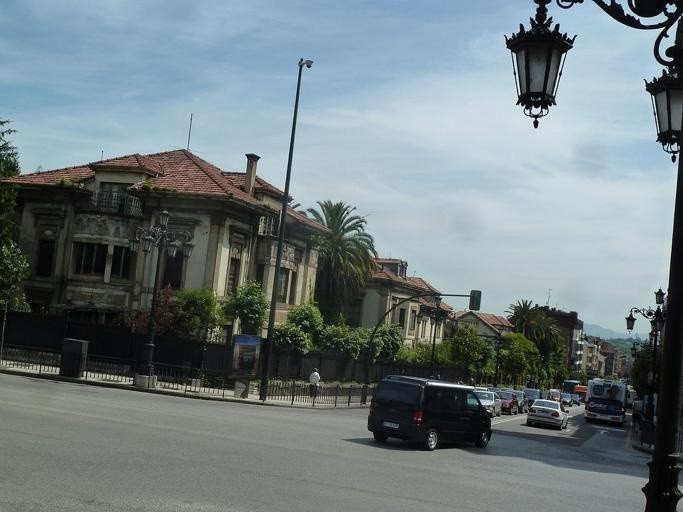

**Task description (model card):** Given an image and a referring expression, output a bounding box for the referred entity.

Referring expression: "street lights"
[133,210,183,385]
[256,54,317,401]
[623,281,670,451]
[502,0,683,511]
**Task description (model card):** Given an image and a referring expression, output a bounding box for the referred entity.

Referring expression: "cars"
[461,375,634,433]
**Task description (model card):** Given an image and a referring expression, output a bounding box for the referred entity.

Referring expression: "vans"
[365,374,493,451]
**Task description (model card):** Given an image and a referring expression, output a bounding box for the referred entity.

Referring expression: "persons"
[308,367,320,398]
